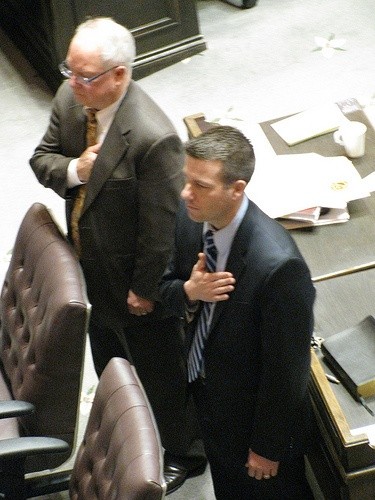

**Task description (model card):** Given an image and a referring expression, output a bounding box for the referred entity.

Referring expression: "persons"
[155,125,316,500]
[28,16,207,496]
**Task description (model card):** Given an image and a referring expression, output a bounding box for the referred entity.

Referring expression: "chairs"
[0,202,167,500]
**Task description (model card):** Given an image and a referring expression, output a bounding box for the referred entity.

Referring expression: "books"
[320,314,375,400]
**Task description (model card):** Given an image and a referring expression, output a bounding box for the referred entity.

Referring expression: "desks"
[181,94,375,500]
[0,0,208,98]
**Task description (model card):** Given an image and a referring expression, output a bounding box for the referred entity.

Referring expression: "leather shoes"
[162,464,205,496]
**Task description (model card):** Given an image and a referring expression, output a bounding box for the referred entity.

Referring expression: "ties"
[71,108,98,248]
[188,231,217,383]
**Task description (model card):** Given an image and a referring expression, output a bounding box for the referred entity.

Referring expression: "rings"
[263,475,270,479]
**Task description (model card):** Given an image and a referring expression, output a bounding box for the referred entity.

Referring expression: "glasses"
[57,62,118,87]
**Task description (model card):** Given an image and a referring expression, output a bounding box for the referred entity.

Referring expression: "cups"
[333,122,367,158]
